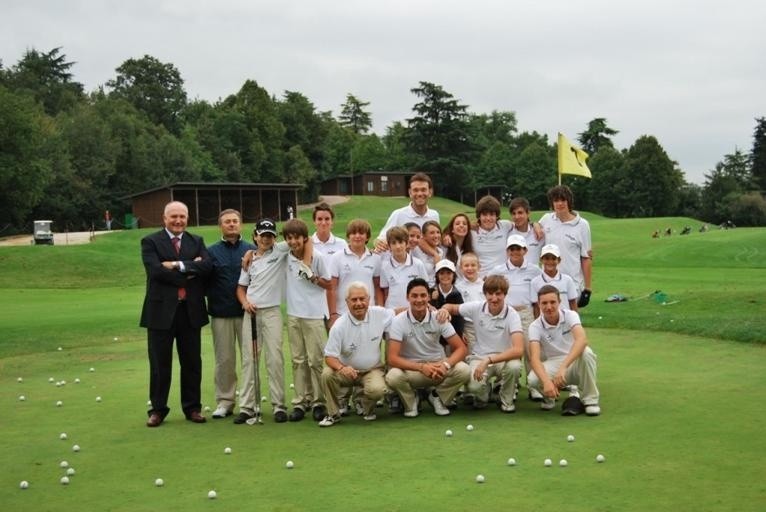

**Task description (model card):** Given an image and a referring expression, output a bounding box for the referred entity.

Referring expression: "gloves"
[577,288,591,308]
[299,262,314,281]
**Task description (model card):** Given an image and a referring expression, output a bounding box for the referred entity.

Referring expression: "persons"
[103,210,113,231]
[652,220,736,238]
[205,209,259,419]
[139,201,213,427]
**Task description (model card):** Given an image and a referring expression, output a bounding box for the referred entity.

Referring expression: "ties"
[172,238,188,302]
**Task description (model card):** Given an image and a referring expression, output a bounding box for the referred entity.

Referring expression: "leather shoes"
[146,413,161,427]
[190,411,205,424]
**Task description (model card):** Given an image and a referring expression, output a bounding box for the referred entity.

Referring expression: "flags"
[558,133,592,179]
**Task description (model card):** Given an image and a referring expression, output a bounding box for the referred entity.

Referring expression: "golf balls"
[59,433,81,485]
[286,461,293,468]
[20,481,29,489]
[467,424,473,430]
[476,474,484,483]
[446,430,452,436]
[57,347,63,351]
[148,400,152,405]
[208,490,216,498]
[225,448,231,454]
[670,320,674,323]
[508,434,606,466]
[598,317,602,319]
[656,312,660,316]
[156,479,164,486]
[17,367,103,408]
[290,383,295,389]
[262,396,267,402]
[114,337,118,341]
[205,406,211,411]
[236,391,239,396]
[662,302,666,305]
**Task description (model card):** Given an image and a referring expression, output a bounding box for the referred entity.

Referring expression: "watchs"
[172,261,177,269]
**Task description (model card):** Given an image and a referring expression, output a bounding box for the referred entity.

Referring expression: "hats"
[561,395,585,417]
[540,243,560,258]
[434,259,456,274]
[256,218,278,237]
[505,234,527,248]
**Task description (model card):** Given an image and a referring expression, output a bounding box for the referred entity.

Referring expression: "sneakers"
[273,411,288,423]
[388,397,402,414]
[561,385,581,397]
[403,389,420,418]
[584,405,601,416]
[540,396,556,410]
[318,407,347,427]
[288,408,304,422]
[312,406,326,420]
[451,385,519,413]
[211,407,230,418]
[232,412,250,424]
[355,401,383,420]
[527,387,544,400]
[427,389,450,416]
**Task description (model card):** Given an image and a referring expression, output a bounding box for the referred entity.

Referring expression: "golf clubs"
[246,311,264,426]
